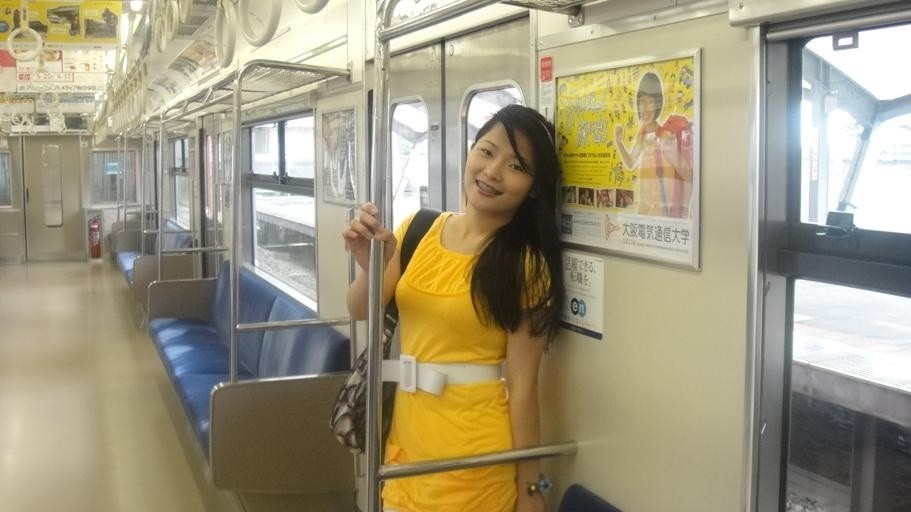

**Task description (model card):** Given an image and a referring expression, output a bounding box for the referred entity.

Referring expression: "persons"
[342,104,564,512]
[615,72,693,218]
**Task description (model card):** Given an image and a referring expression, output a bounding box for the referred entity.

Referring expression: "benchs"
[110,217,197,283]
[144,264,360,504]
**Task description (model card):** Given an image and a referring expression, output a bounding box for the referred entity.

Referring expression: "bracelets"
[516,474,553,496]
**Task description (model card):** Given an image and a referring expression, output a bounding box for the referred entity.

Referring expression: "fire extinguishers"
[88,215,101,257]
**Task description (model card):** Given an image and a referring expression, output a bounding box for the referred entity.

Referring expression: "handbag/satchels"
[327,314,398,455]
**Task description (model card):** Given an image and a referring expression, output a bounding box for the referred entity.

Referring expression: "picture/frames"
[550,47,703,271]
